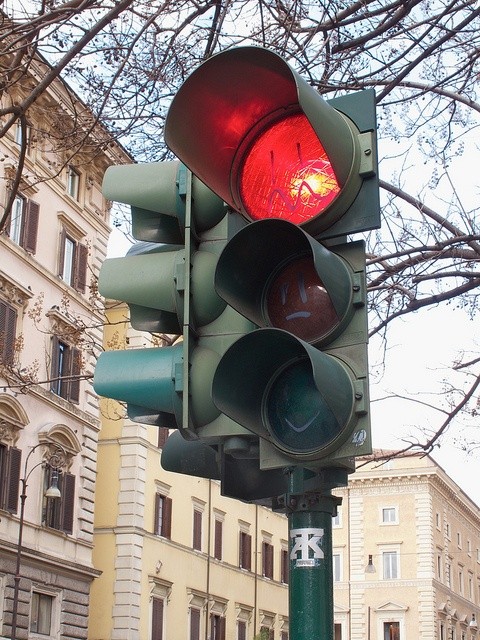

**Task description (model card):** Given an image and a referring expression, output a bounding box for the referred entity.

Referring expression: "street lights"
[9,442,65,639]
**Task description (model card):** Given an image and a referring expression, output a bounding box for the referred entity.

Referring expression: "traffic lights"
[96,165,216,417]
[168,43,370,458]
